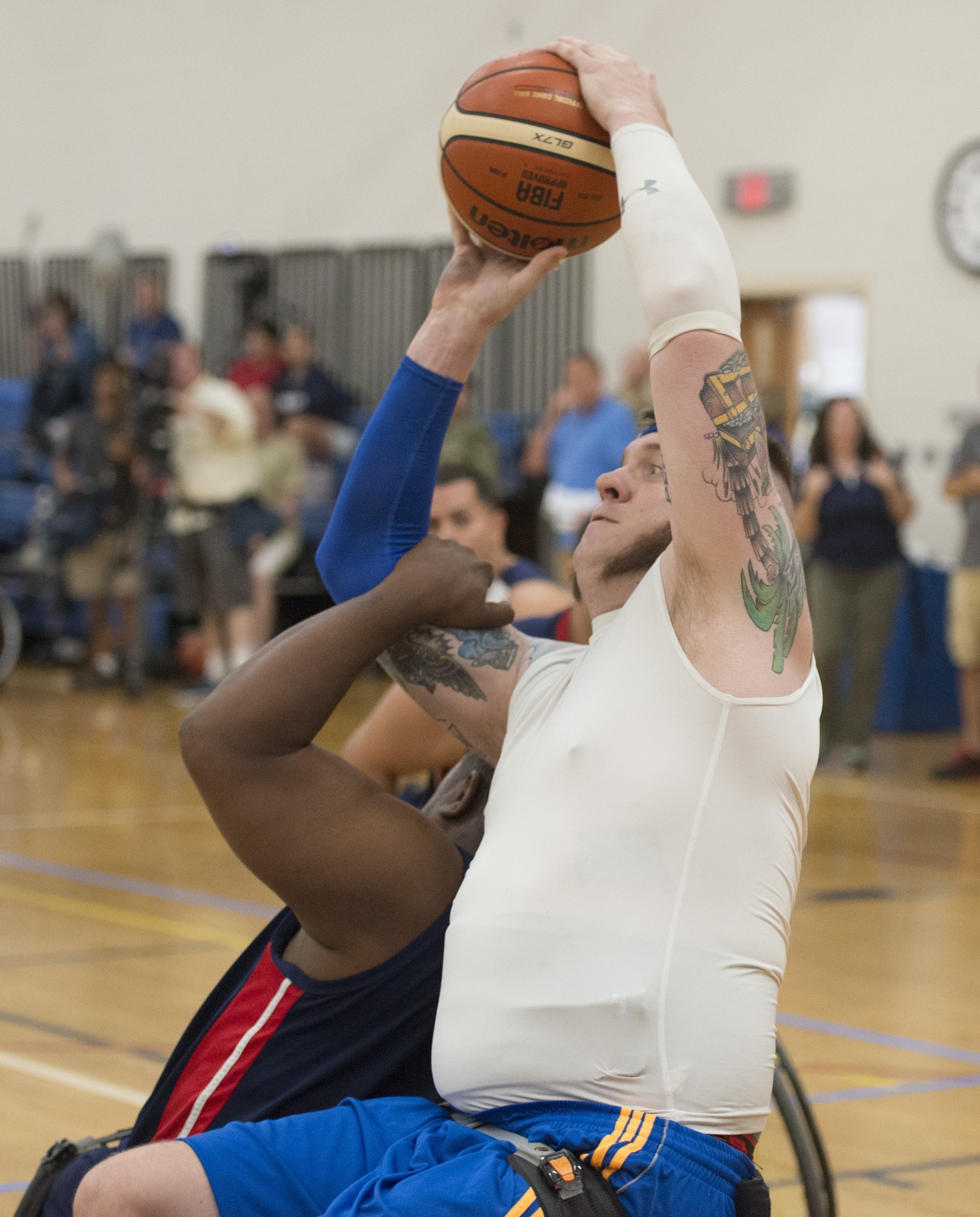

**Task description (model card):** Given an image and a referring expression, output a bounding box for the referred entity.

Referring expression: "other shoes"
[929,751,978,777]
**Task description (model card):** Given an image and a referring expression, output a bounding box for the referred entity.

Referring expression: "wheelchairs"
[15,1035,838,1217]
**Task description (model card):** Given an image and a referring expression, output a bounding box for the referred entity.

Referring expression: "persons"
[71,40,826,1217]
[132,534,514,1150]
[339,461,593,809]
[4,262,979,786]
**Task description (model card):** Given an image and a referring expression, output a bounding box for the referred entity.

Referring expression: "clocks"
[936,139,979,275]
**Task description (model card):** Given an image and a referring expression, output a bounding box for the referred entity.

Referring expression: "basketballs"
[437,48,621,260]
